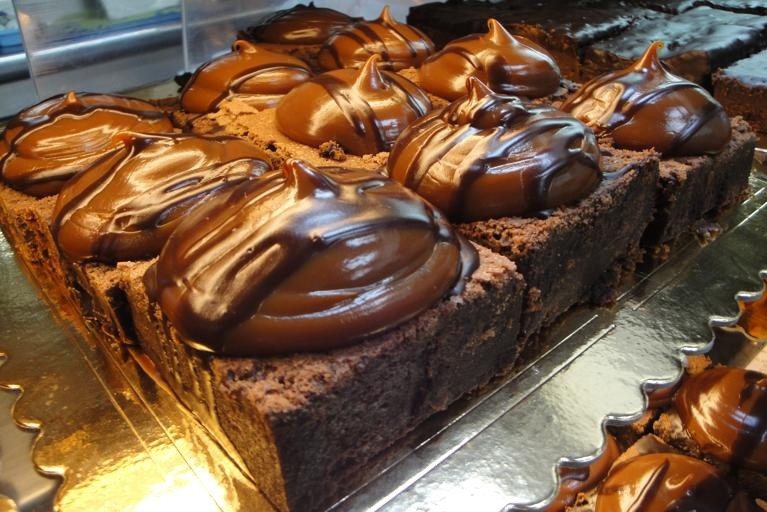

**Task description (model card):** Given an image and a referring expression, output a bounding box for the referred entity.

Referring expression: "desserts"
[0,1,767,512]
